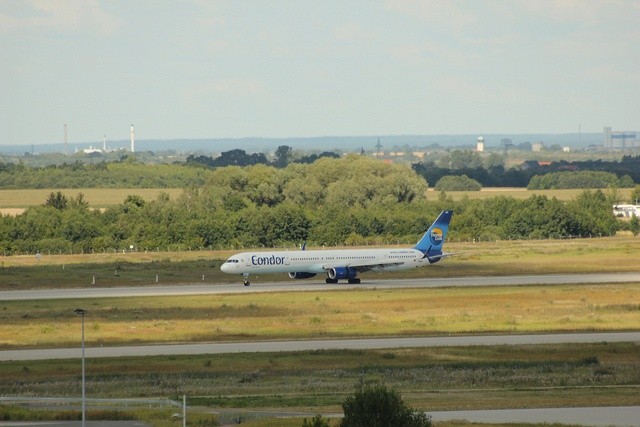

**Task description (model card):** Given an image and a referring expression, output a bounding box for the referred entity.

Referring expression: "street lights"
[74,309,87,427]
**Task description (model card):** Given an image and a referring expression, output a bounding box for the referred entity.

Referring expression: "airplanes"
[220,210,468,286]
